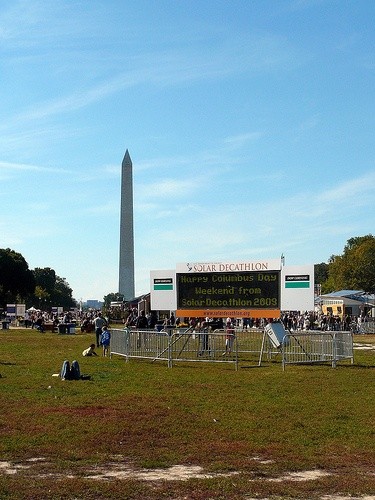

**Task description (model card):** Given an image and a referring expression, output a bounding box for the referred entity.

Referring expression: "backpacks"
[102,332,110,344]
[138,319,145,329]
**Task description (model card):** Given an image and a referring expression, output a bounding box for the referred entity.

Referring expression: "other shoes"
[222,353,225,356]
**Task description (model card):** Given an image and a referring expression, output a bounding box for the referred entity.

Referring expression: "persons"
[124,313,137,347]
[81,344,99,357]
[9,305,157,333]
[133,310,149,348]
[197,321,211,356]
[60,360,80,381]
[161,310,369,334]
[222,322,235,355]
[92,312,107,348]
[99,326,111,357]
